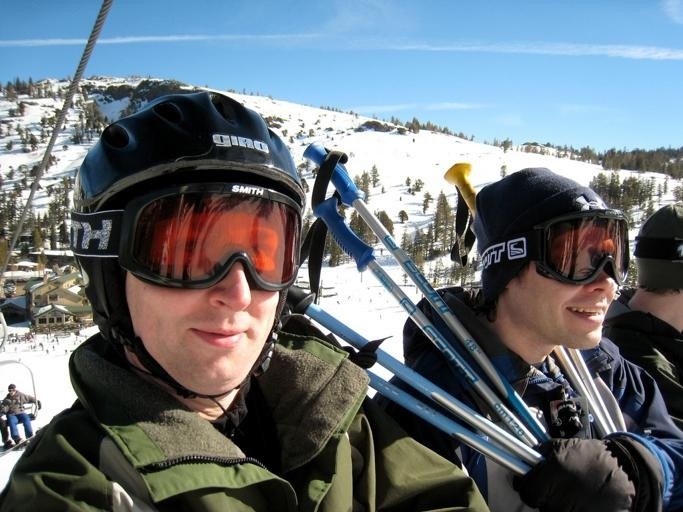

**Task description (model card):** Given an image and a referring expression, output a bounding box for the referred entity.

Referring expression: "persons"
[0,412,14,449]
[0,383,42,446]
[30,333,81,354]
[371,165,683,512]
[1,87,493,512]
[601,199,683,429]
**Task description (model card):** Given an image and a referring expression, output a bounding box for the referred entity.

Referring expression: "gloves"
[512,434,663,512]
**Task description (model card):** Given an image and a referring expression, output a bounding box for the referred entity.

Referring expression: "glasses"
[69,184,303,292]
[481,209,630,286]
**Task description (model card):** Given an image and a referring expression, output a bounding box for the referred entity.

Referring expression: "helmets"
[73,89,306,344]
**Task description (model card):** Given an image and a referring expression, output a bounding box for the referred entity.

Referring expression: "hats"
[634,202,683,289]
[473,167,609,312]
